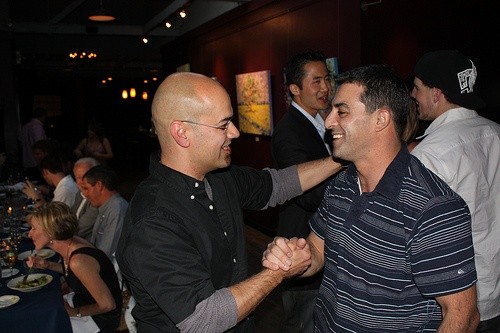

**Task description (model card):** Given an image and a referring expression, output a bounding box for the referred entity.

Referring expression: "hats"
[415,50,486,109]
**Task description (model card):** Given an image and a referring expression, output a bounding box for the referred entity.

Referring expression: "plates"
[21,231,28,237]
[2,269,19,278]
[18,248,55,261]
[7,273,53,292]
[0,295,19,308]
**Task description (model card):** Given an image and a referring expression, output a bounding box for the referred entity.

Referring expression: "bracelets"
[76,306,81,317]
[32,196,42,204]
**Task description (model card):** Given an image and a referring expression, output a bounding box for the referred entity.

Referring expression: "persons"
[269,50,338,333]
[405,98,423,152]
[115,72,343,333]
[260,64,480,333]
[410,53,500,333]
[0,106,130,333]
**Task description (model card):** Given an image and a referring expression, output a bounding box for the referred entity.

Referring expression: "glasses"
[181,119,232,134]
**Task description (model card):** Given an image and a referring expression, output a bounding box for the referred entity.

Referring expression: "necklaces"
[63,242,71,276]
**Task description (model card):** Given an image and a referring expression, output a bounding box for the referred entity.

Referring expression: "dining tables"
[0,188,73,333]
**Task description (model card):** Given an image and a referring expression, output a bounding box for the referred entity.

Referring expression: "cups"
[4,253,16,269]
